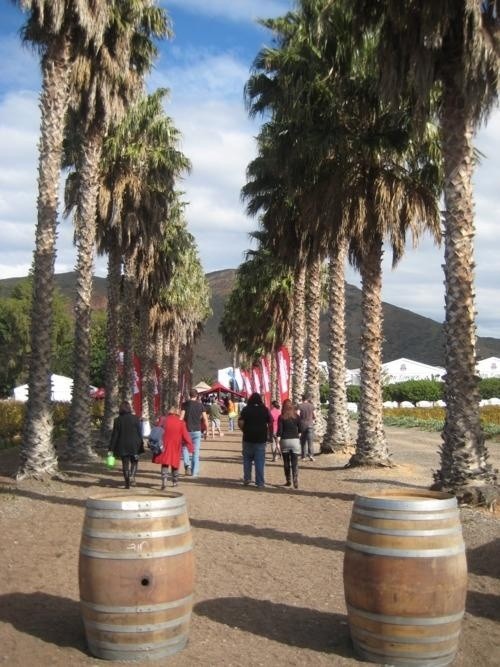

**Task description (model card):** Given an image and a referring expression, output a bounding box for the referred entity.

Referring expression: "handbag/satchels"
[106,454,116,469]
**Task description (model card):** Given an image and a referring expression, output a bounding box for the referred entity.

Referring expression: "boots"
[123,462,198,499]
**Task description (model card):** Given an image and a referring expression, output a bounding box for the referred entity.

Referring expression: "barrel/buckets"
[344,486,468,667]
[78,491,194,662]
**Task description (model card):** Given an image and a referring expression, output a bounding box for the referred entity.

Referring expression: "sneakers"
[244,454,315,491]
[204,428,235,441]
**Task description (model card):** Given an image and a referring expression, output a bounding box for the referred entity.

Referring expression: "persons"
[108,389,316,490]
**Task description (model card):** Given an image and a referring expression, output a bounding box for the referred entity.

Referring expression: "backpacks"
[147,416,165,456]
